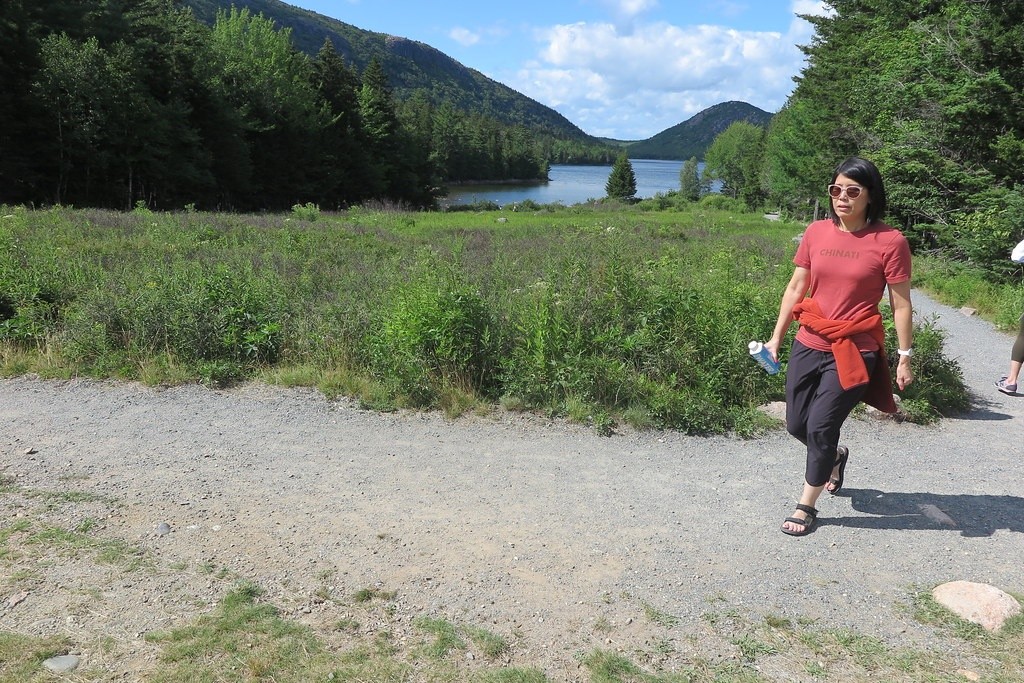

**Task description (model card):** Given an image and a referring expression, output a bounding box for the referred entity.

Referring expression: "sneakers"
[995,377,1017,393]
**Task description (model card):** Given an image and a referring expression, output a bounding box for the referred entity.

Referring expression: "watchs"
[897,348,913,358]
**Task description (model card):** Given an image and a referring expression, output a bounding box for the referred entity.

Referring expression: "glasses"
[827,185,866,199]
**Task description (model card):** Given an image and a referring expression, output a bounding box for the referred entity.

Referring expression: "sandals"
[781,503,819,535]
[827,445,849,494]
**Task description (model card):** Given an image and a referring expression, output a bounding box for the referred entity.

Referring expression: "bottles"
[748,341,781,375]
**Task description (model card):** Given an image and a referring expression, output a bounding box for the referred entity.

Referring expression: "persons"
[761,155,917,536]
[995,238,1024,393]
